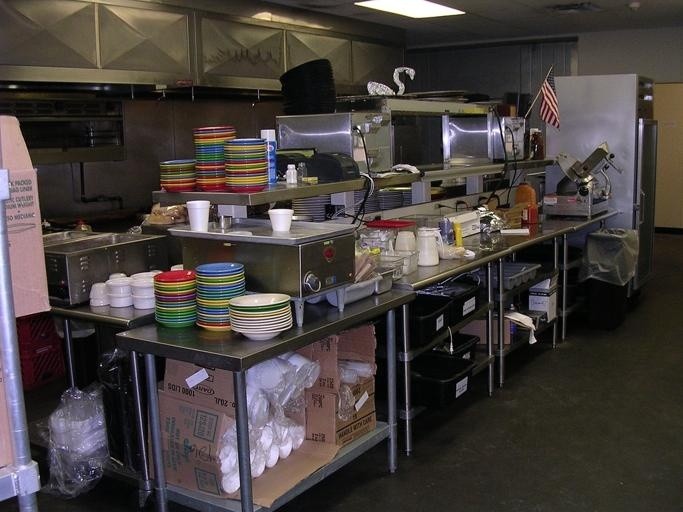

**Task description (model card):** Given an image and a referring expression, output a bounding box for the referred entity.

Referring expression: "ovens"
[0,88,128,164]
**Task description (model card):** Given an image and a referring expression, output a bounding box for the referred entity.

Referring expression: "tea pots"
[415,227,445,267]
[396,231,415,251]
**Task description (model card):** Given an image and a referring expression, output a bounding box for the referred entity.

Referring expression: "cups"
[338,357,375,384]
[268,209,295,232]
[186,200,212,232]
[219,352,321,494]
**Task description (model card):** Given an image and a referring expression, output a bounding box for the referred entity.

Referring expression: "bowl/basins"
[88,269,162,310]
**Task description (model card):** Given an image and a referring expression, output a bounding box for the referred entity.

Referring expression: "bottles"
[285,164,298,184]
[440,217,454,247]
[258,129,277,185]
[297,162,308,185]
[514,182,538,224]
[530,131,545,161]
[509,303,517,335]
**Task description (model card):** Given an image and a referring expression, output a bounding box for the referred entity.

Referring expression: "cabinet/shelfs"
[25,298,156,511]
[115,288,414,511]
[0,169,45,512]
[390,209,624,458]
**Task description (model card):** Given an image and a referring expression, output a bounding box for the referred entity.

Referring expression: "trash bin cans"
[586,227,639,331]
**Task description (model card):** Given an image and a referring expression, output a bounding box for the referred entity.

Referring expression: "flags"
[539,65,561,131]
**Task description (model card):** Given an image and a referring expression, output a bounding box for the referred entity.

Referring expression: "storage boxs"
[1,115,55,318]
[0,327,21,470]
[148,318,377,508]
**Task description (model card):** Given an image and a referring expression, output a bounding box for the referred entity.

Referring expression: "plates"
[291,192,331,222]
[278,56,336,116]
[158,124,269,195]
[353,187,412,215]
[152,261,294,343]
